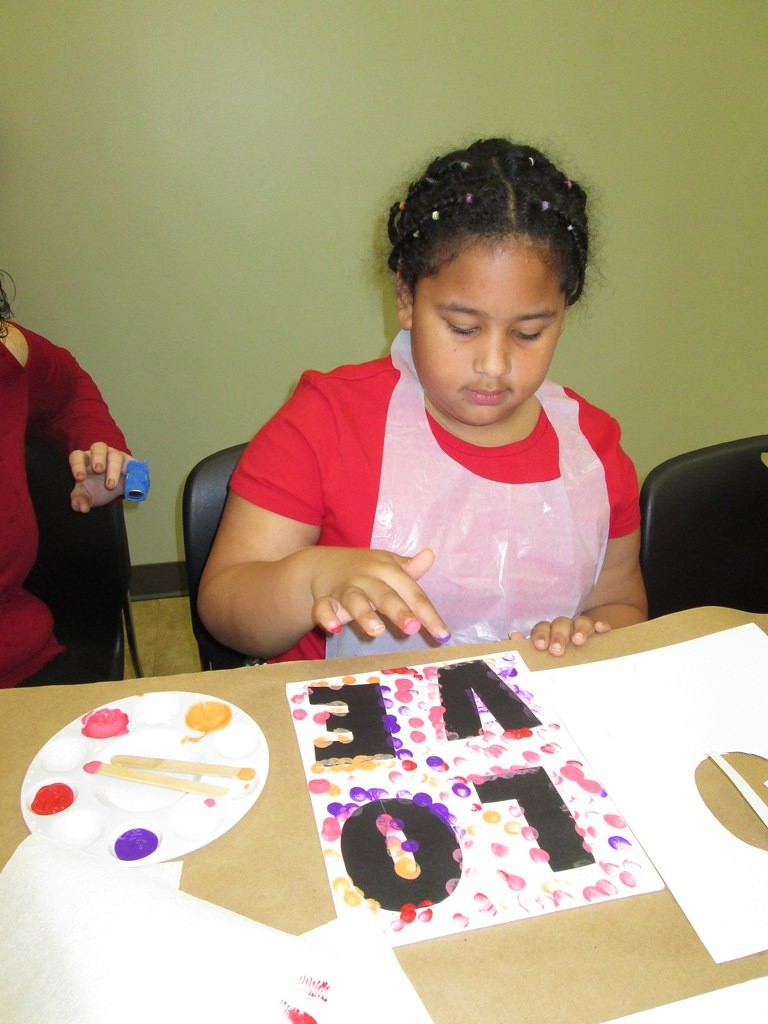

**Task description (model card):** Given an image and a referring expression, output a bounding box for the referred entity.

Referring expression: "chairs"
[182,442,249,671]
[638,434,767,621]
[17,429,146,688]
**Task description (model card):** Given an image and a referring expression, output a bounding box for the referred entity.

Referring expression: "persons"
[0,264,153,688]
[199,139,649,655]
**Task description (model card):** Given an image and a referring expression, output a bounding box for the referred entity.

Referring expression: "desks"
[0,606,767,1024]
